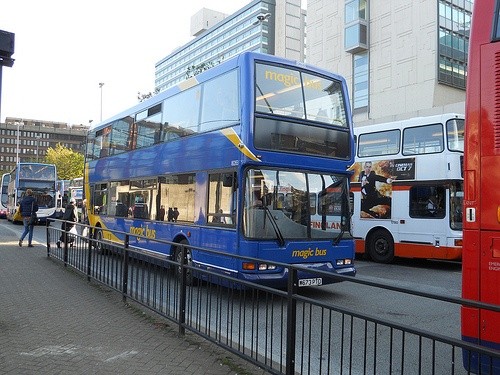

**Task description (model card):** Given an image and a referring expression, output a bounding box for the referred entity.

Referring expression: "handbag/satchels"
[28,215,39,226]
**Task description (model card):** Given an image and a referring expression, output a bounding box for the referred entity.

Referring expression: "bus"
[0,163,57,225]
[304,111,467,264]
[56,177,84,219]
[78,50,357,292]
[456,0,499,375]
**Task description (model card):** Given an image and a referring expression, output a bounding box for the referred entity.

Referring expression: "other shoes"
[372,210,379,218]
[70,243,76,247]
[28,244,34,247]
[56,241,62,248]
[19,240,22,247]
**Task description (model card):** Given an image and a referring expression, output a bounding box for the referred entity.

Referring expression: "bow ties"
[364,175,367,177]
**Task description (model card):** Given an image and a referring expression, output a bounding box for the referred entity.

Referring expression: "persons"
[220,209,226,224]
[81,199,89,236]
[19,189,38,247]
[111,199,129,233]
[56,199,78,248]
[199,207,205,225]
[167,207,179,222]
[159,205,165,221]
[424,191,439,215]
[358,162,394,218]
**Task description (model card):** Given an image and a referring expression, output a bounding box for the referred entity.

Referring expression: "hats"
[70,198,75,202]
